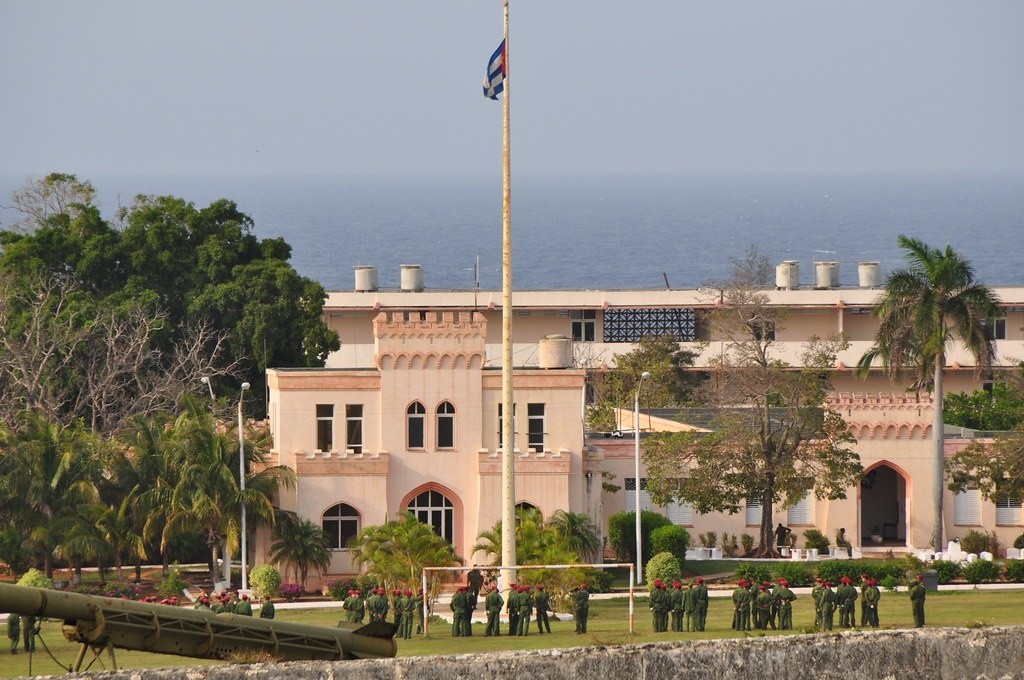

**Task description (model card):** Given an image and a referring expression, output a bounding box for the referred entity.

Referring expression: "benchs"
[685,546,722,560]
[777,545,818,561]
[828,546,861,559]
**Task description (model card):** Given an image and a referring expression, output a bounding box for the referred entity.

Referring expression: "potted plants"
[869,525,883,544]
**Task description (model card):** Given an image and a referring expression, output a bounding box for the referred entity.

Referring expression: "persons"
[343,588,431,640]
[467,564,483,609]
[107,589,274,620]
[6,612,21,655]
[21,614,38,652]
[649,573,925,633]
[1013,532,1024,549]
[448,583,589,636]
[774,523,791,555]
[836,528,853,560]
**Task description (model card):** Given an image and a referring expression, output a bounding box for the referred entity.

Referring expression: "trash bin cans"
[921,570,939,591]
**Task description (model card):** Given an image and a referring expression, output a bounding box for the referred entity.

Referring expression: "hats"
[750,578,755,583]
[778,577,784,582]
[916,575,923,579]
[816,578,822,581]
[842,576,849,583]
[782,581,789,587]
[759,586,765,590]
[866,580,872,586]
[654,580,662,588]
[673,581,678,585]
[661,585,666,590]
[738,580,743,586]
[910,579,918,584]
[780,581,784,585]
[764,581,769,587]
[675,583,681,589]
[846,578,852,584]
[871,578,877,583]
[123,583,588,606]
[697,578,703,583]
[743,583,748,588]
[742,579,746,583]
[862,574,869,579]
[821,580,827,585]
[688,582,694,587]
[825,582,831,586]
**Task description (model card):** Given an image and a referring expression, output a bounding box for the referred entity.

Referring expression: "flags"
[484,38,507,100]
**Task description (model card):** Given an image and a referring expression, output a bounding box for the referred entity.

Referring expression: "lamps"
[585,472,592,479]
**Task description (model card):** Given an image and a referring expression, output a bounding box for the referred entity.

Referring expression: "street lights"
[202,376,231,590]
[634,371,651,585]
[236,382,251,590]
